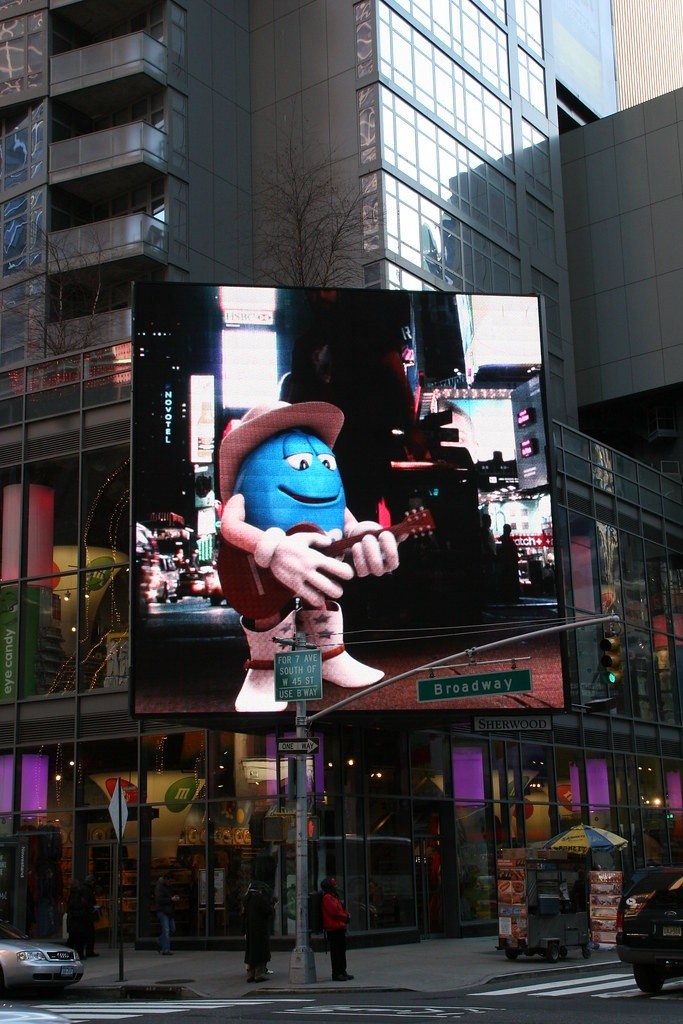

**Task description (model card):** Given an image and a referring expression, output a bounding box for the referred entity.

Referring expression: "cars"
[0,916,84,998]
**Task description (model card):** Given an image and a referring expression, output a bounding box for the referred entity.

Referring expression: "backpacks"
[308,891,324,930]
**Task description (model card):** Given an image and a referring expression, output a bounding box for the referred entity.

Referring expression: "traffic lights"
[306,815,321,842]
[601,632,624,687]
[262,815,289,842]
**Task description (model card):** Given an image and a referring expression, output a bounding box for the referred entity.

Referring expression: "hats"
[321,877,339,898]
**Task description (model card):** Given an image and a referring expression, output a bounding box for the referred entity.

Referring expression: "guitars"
[217,506,436,619]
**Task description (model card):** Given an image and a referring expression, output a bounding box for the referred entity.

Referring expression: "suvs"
[615,865,683,995]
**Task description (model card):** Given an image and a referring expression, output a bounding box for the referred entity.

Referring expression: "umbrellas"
[542,822,628,854]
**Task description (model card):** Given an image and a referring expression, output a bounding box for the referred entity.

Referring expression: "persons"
[320,877,354,981]
[574,871,586,909]
[243,881,279,982]
[66,883,102,960]
[154,883,180,955]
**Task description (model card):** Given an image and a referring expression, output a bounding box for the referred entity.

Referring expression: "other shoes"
[155,943,161,953]
[80,956,86,960]
[255,976,268,983]
[266,970,273,974]
[86,953,99,957]
[162,952,173,955]
[247,977,255,983]
[332,972,354,981]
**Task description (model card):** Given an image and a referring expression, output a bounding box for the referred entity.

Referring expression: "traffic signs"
[275,737,320,755]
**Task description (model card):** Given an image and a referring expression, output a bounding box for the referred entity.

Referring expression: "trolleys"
[495,822,630,963]
